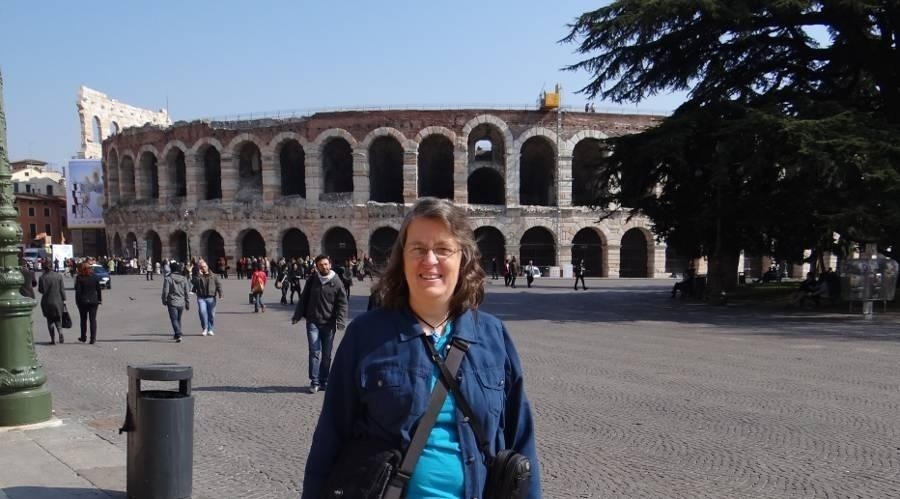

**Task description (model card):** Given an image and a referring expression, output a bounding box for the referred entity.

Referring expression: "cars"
[83,264,112,290]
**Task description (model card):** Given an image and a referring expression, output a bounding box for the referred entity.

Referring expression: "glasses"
[403,244,463,258]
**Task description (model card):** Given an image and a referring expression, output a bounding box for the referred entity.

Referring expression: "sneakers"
[48,340,55,344]
[174,335,183,342]
[320,383,328,390]
[59,338,64,343]
[208,331,214,336]
[201,329,207,336]
[309,384,319,394]
[262,304,265,312]
[90,338,96,344]
[78,337,86,342]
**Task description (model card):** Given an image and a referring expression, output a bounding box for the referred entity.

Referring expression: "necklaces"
[412,311,454,342]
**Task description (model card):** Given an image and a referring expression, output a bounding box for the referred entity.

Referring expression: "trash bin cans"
[126,363,194,498]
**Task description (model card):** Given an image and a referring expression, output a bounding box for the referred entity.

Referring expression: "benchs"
[798,293,831,311]
[682,276,706,296]
[762,272,785,284]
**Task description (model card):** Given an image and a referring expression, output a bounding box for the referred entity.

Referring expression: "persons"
[192,260,222,335]
[572,258,588,290]
[20,259,37,299]
[162,264,191,342]
[74,264,101,344]
[236,253,374,313]
[752,266,783,283]
[491,256,534,288]
[39,260,66,344]
[291,255,347,392]
[304,197,543,499]
[41,253,139,277]
[792,271,812,303]
[146,255,228,280]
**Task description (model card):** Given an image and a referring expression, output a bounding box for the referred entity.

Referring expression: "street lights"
[182,209,194,266]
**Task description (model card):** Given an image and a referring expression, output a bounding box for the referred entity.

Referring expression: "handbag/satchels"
[249,294,256,304]
[277,281,282,289]
[482,449,531,499]
[253,283,264,294]
[320,441,408,499]
[62,309,72,328]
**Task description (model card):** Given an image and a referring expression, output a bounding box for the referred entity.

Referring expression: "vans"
[22,247,50,270]
